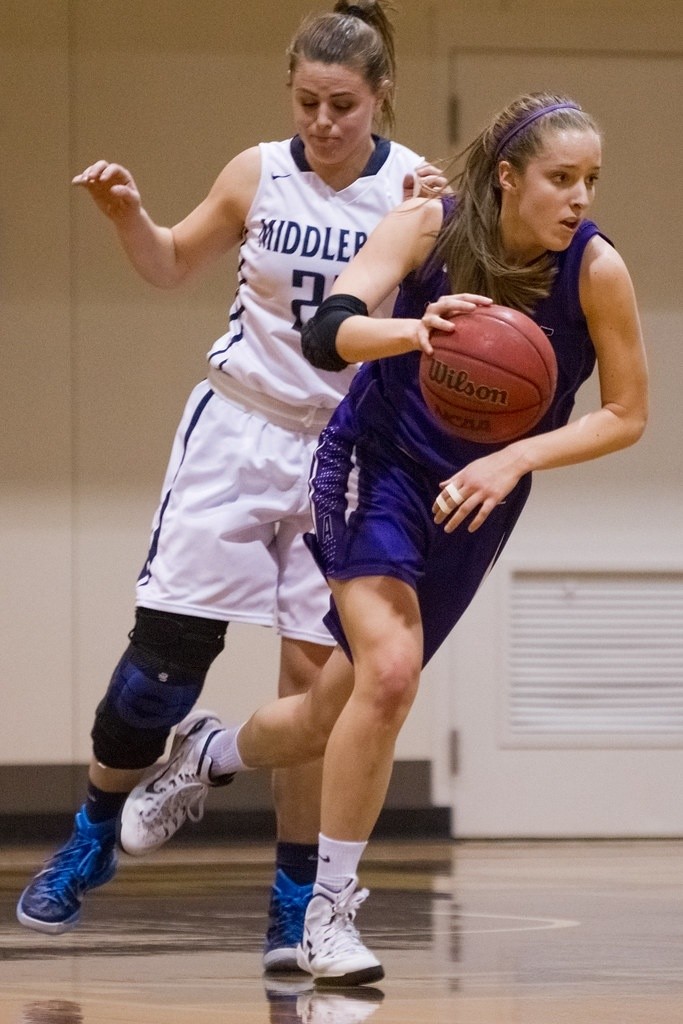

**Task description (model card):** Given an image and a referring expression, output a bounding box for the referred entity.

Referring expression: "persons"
[115,91,649,987]
[15,0,452,971]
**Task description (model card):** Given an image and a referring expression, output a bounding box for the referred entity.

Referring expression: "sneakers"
[16,803,118,933]
[297,878,387,986]
[262,866,319,972]
[114,709,235,858]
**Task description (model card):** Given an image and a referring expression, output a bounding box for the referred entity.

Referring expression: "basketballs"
[414,298,560,446]
